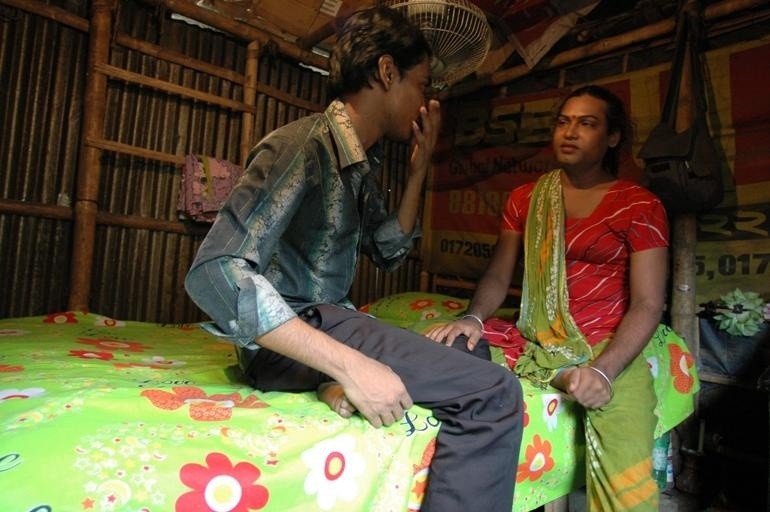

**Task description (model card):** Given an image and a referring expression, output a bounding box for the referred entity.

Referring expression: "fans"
[378,0,492,91]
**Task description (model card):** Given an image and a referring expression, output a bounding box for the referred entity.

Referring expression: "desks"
[699,367,769,458]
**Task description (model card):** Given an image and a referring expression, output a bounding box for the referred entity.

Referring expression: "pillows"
[356,291,466,324]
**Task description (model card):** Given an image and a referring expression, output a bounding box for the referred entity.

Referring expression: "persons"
[184,5,525,512]
[419,81,675,511]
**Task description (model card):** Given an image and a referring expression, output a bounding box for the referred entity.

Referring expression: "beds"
[1,211,696,511]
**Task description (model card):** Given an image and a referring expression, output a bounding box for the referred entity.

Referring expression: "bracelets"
[459,313,486,336]
[587,363,614,391]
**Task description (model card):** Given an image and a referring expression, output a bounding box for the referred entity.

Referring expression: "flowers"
[708,285,770,340]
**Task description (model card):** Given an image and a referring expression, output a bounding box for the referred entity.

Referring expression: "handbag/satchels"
[636,117,738,216]
